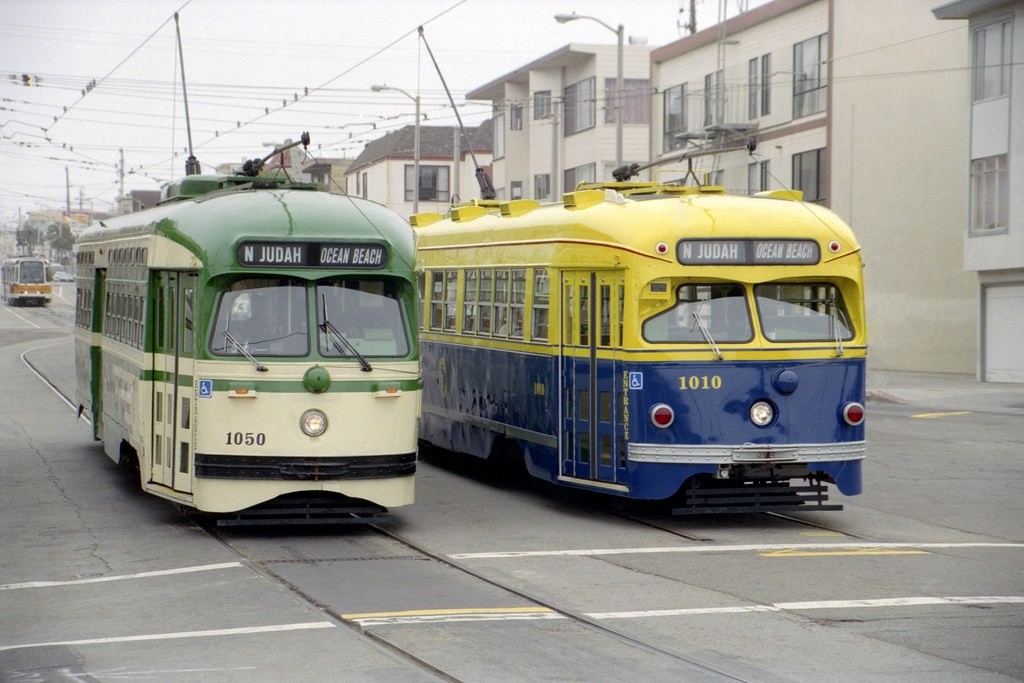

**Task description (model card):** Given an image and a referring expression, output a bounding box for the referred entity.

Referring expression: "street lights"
[372,85,420,216]
[554,15,624,168]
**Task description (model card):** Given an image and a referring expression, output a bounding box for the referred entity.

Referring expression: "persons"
[237,294,277,339]
[498,305,540,339]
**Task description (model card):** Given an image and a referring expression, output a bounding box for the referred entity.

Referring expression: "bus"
[70,11,419,532]
[2,206,51,307]
[410,26,868,518]
